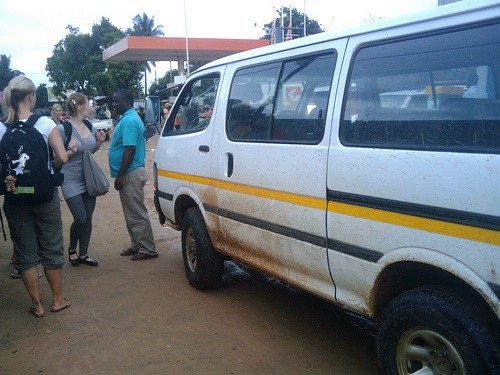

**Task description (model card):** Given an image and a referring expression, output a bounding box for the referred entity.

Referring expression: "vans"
[153,1,500,375]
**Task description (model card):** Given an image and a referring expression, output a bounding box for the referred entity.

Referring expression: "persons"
[1,76,71,318]
[91,92,216,141]
[107,89,159,261]
[59,92,107,267]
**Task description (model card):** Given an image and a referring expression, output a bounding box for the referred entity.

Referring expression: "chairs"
[308,91,328,131]
[231,86,273,139]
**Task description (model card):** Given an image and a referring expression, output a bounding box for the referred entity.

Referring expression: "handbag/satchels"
[81,149,109,197]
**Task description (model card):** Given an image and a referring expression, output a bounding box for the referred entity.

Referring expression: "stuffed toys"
[4,175,16,193]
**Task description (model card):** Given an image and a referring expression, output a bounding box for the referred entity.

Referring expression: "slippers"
[50,297,70,311]
[31,306,44,316]
[130,252,158,260]
[120,245,139,256]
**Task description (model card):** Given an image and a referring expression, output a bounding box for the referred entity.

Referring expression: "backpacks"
[0,114,64,205]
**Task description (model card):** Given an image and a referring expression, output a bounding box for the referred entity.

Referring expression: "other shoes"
[79,256,98,266]
[68,247,80,265]
[11,270,43,278]
